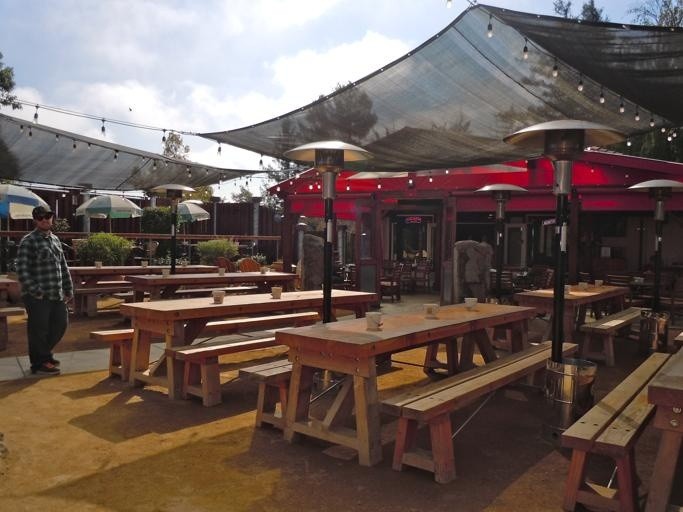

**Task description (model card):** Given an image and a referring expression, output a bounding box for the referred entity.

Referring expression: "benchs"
[382,341,579,484]
[75,286,132,317]
[163,335,292,406]
[559,352,677,512]
[0,307,25,351]
[238,336,460,433]
[581,306,652,366]
[111,286,259,325]
[89,310,319,381]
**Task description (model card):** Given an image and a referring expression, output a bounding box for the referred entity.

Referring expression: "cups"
[213,291,225,304]
[564,285,571,299]
[595,280,603,288]
[260,267,266,274]
[578,282,588,291]
[141,261,148,268]
[95,261,102,269]
[365,311,382,331]
[464,297,477,311]
[182,262,187,268]
[424,304,438,319]
[218,268,225,276]
[272,287,282,299]
[162,270,170,278]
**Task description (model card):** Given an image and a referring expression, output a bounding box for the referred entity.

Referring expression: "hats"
[32,205,55,218]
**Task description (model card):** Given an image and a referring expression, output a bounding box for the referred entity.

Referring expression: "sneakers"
[30,362,60,375]
[47,353,60,365]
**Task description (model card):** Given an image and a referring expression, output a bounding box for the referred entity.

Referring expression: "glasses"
[35,215,51,221]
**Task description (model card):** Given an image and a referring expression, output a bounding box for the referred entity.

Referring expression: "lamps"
[485,12,680,139]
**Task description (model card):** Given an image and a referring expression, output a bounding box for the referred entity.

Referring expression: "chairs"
[377,265,402,304]
[515,267,553,291]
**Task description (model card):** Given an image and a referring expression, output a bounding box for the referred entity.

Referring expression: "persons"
[17,206,75,375]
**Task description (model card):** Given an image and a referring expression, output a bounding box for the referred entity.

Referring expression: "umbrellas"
[0,184,50,243]
[75,193,142,236]
[168,200,211,237]
